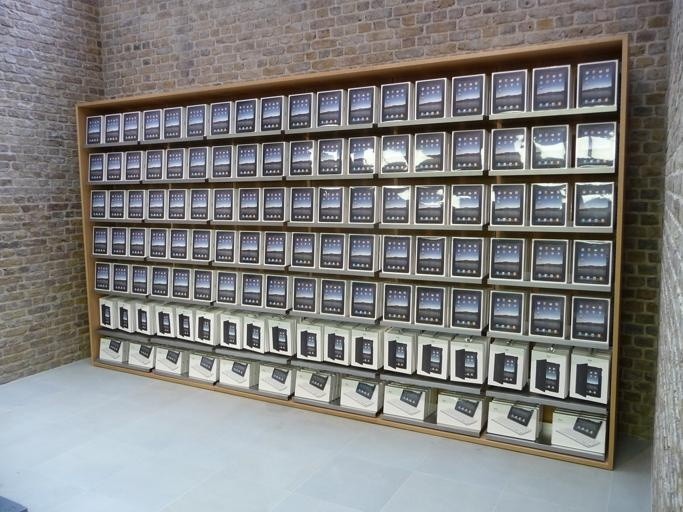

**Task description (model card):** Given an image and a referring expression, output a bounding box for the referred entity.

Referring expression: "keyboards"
[556,428,600,448]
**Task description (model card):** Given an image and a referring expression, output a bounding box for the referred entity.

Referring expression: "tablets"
[85,59,617,438]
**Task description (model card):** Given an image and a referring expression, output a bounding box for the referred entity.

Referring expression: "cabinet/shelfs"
[74,30,633,471]
